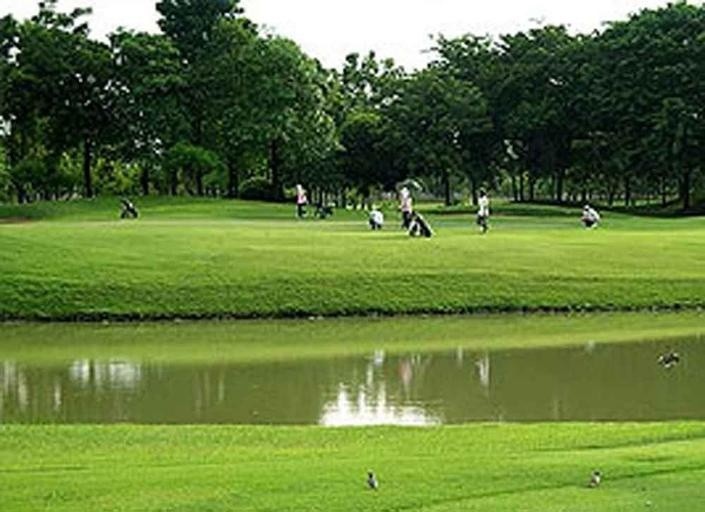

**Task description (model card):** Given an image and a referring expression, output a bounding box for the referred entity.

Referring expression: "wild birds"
[367,470,377,489]
[588,470,602,488]
[657,349,681,369]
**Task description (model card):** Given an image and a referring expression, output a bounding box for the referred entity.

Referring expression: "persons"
[580,204,603,229]
[117,198,139,220]
[368,207,384,229]
[398,186,414,230]
[295,183,310,219]
[476,188,491,234]
[404,212,433,238]
